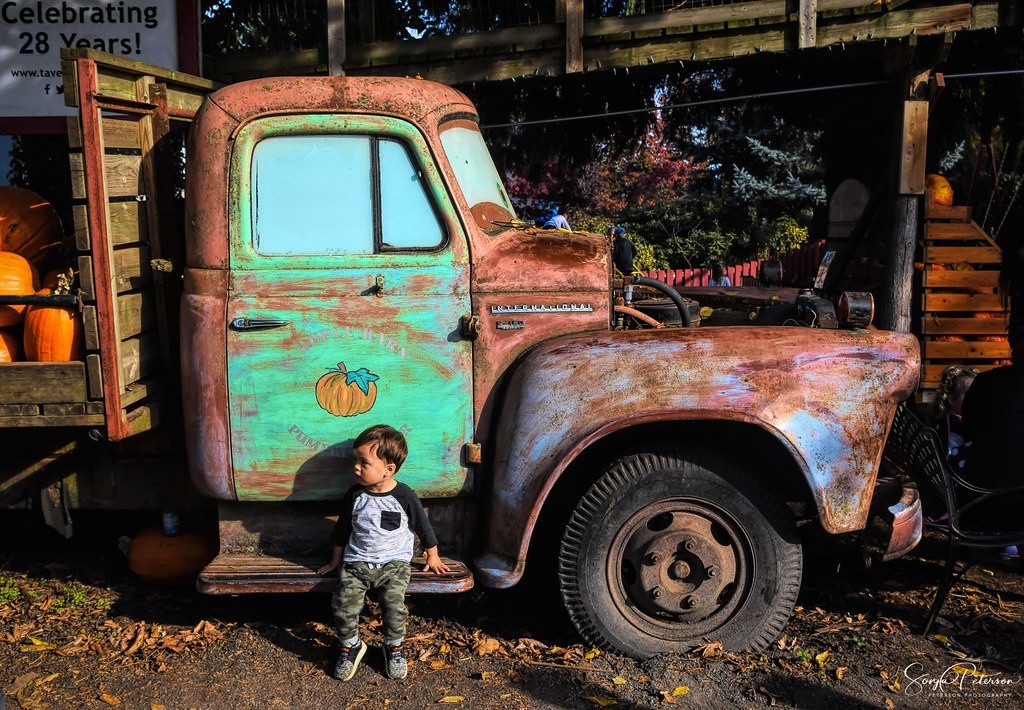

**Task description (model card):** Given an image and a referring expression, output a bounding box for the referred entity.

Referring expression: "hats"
[615,228,625,235]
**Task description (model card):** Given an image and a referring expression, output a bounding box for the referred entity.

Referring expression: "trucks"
[0,47,923,660]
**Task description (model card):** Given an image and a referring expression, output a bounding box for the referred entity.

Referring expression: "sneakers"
[382,642,407,680]
[334,639,367,682]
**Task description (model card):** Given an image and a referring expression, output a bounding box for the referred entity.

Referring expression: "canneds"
[162,511,180,535]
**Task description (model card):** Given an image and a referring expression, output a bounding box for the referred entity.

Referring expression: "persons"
[317,424,450,682]
[612,227,637,278]
[542,207,571,233]
[709,261,730,287]
[922,365,1020,558]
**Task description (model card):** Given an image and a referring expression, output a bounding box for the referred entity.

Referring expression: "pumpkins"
[915,262,1011,366]
[117,529,217,579]
[0,186,84,363]
[925,174,953,207]
